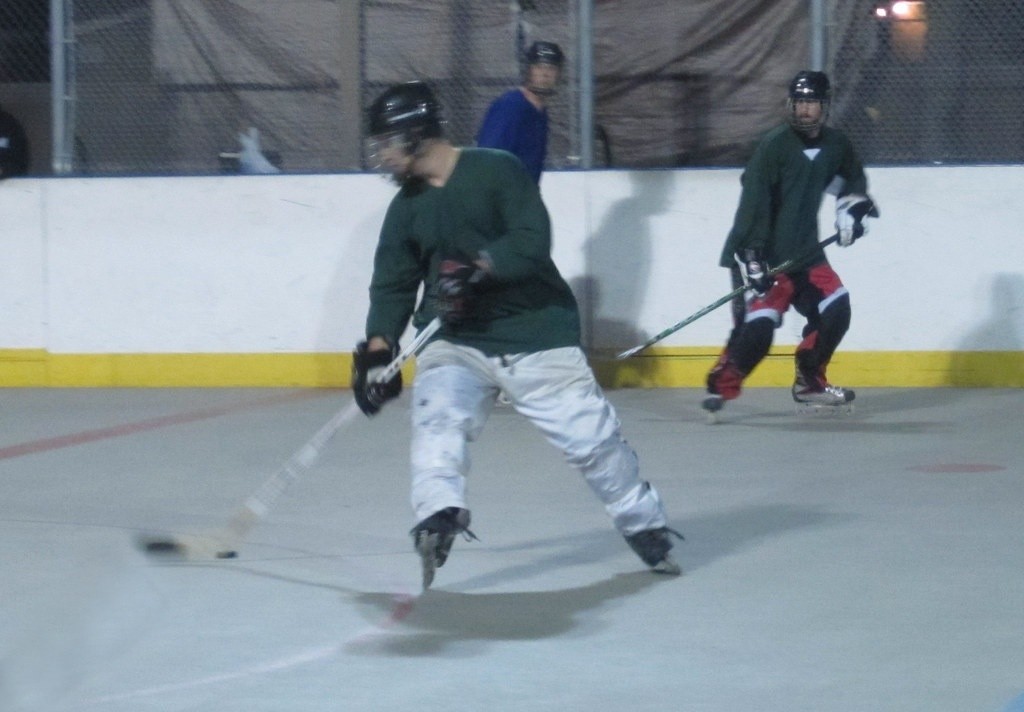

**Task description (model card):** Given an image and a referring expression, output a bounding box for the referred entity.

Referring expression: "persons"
[0,103,29,180]
[702,70,879,423]
[468,41,565,183]
[352,79,681,590]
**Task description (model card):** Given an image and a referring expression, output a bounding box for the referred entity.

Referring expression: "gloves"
[734,231,776,298]
[352,341,403,420]
[436,257,496,304]
[834,189,880,247]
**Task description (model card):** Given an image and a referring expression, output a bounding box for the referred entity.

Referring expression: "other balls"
[216,550,235,560]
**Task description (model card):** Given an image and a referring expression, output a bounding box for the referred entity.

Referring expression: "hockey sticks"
[616,233,837,360]
[131,314,443,563]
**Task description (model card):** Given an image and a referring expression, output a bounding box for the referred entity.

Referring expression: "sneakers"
[700,393,729,428]
[792,383,856,418]
[409,507,482,591]
[623,526,685,576]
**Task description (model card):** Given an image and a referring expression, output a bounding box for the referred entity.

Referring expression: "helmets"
[364,80,444,195]
[785,70,833,133]
[527,42,564,94]
